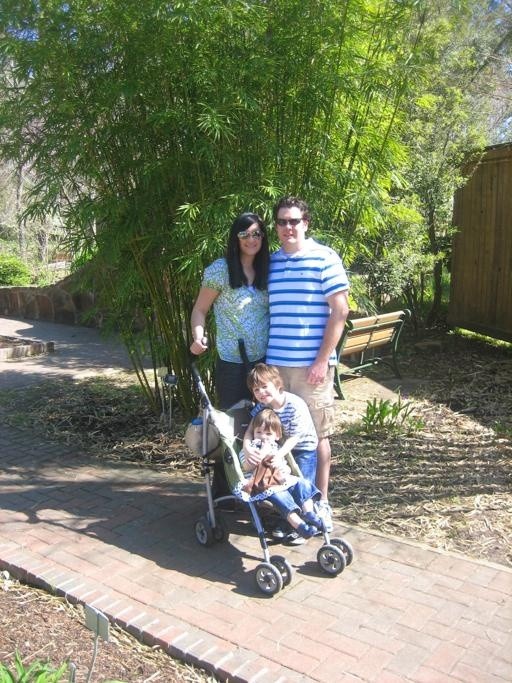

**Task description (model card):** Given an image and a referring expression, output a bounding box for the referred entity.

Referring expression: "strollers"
[188,342,354,596]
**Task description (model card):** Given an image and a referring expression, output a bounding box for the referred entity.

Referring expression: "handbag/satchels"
[185,417,223,458]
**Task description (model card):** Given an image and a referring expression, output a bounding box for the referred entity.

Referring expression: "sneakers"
[316,500,332,532]
[212,494,234,508]
[272,512,322,546]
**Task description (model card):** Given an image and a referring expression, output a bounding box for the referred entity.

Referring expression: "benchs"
[332,308,413,400]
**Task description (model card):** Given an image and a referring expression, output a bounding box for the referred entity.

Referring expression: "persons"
[188,212,271,514]
[263,195,351,534]
[238,407,323,539]
[246,362,320,546]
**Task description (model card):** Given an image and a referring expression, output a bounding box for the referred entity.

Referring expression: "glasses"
[237,231,264,241]
[277,218,303,226]
